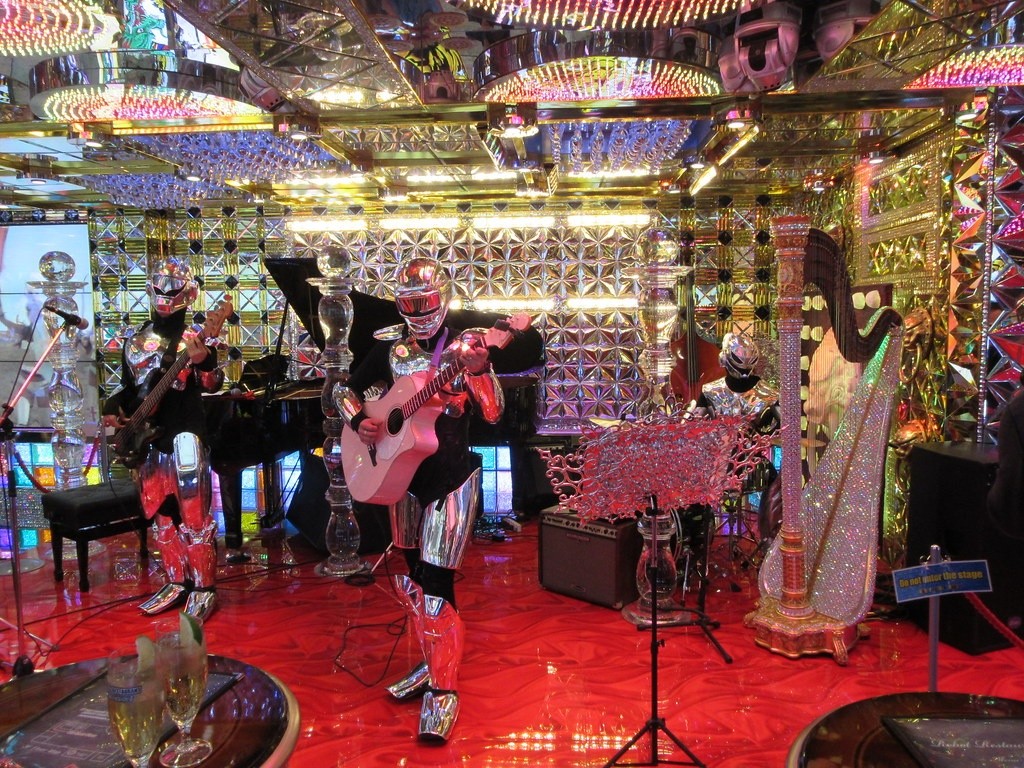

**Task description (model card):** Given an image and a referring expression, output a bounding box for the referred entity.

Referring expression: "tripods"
[536,412,787,768]
[0,321,72,684]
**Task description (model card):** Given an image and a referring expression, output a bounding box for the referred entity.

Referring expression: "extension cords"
[502,518,521,532]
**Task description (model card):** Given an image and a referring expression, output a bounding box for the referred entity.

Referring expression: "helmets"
[145,256,199,318]
[394,257,456,340]
[717,331,759,381]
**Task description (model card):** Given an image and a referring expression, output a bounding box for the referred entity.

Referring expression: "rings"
[468,357,472,360]
[106,423,109,425]
[364,431,367,435]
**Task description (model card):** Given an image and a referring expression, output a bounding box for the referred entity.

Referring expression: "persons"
[681,332,780,562]
[100,260,224,626]
[330,258,503,742]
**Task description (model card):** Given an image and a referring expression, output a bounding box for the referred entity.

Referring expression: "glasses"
[396,291,441,318]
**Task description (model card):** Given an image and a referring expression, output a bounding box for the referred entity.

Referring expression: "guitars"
[113,293,235,470]
[339,310,533,506]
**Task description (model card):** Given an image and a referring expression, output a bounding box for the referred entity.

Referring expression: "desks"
[0,654,302,768]
[787,692,1024,768]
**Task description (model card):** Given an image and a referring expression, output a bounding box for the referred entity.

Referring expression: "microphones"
[45,306,89,330]
[221,388,241,398]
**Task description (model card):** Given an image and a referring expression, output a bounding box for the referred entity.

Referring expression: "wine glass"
[152,616,213,768]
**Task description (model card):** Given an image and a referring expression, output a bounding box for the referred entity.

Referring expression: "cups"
[106,643,166,768]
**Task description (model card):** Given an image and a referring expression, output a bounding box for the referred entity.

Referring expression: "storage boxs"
[538,499,645,610]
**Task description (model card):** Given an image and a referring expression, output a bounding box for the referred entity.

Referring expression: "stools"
[40,477,154,592]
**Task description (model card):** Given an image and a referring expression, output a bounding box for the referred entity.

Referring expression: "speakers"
[539,505,643,610]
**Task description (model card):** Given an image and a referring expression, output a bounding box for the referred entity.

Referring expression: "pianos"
[101,254,545,550]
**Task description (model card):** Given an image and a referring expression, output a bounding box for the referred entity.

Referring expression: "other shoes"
[135,582,187,616]
[385,661,434,703]
[183,587,218,625]
[417,684,462,744]
